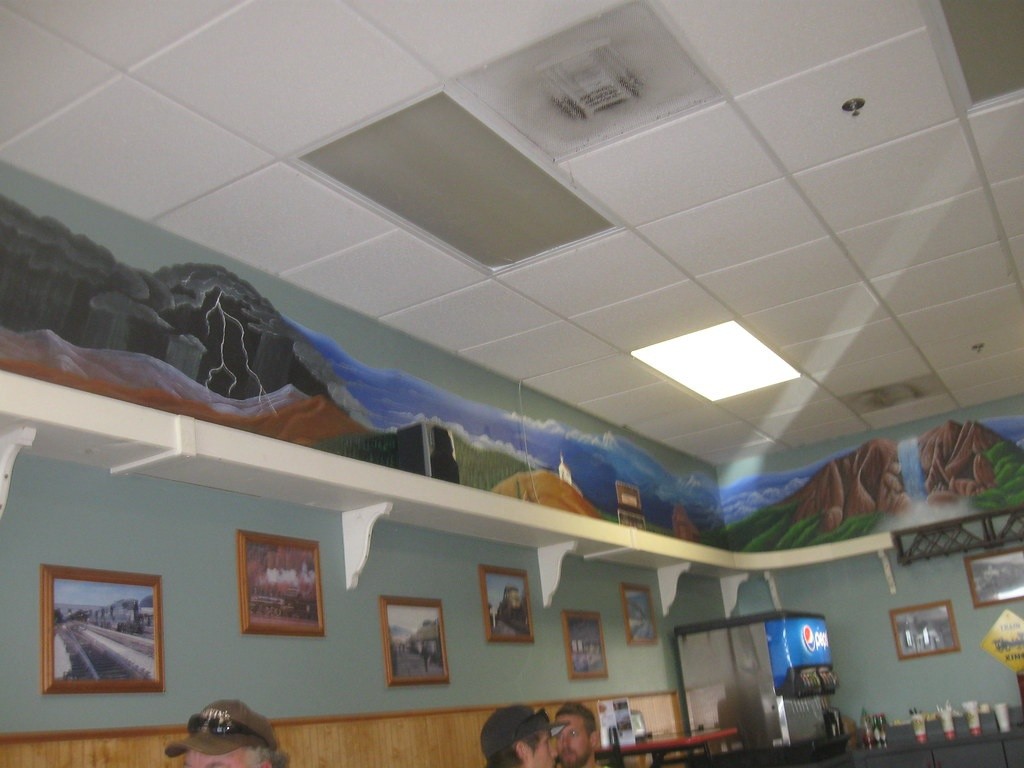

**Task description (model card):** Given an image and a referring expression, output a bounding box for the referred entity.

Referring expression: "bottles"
[861,712,887,749]
[608,726,624,768]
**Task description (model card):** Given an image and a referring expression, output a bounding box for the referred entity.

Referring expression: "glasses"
[187,714,269,747]
[515,706,549,736]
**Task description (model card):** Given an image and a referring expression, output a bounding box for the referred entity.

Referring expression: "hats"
[164,699,277,757]
[480,705,570,758]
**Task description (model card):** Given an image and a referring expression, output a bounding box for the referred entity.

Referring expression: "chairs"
[648,724,715,768]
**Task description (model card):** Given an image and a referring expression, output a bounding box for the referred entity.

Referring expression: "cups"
[911,714,927,744]
[994,703,1011,733]
[939,711,956,740]
[966,710,981,736]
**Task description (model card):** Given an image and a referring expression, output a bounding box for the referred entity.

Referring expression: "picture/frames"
[479,564,535,644]
[890,598,961,660]
[963,547,1024,609]
[379,594,452,687]
[619,582,659,646]
[237,528,325,637]
[38,564,166,695]
[561,609,609,679]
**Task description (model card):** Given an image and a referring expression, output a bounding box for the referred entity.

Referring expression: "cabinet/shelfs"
[811,705,1024,768]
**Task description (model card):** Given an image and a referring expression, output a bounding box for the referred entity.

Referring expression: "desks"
[594,727,737,768]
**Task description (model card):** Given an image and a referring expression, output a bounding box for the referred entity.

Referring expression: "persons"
[554,702,610,768]
[481,705,570,768]
[166,699,289,768]
